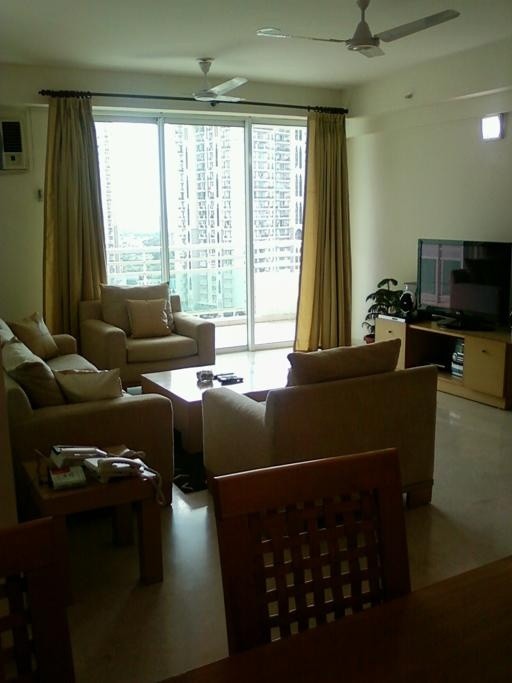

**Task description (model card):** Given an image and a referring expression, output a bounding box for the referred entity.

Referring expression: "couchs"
[0,332,176,524]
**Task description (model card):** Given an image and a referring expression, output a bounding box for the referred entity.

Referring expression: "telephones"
[49,444,110,469]
[84,457,149,484]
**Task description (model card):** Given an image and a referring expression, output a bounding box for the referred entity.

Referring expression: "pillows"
[51,367,125,403]
[124,298,171,339]
[0,316,15,345]
[98,279,175,337]
[2,311,61,360]
[1,335,66,406]
[284,337,402,387]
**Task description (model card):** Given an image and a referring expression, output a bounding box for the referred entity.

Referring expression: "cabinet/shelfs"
[374,317,512,410]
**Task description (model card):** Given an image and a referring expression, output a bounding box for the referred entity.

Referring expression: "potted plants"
[361,278,403,344]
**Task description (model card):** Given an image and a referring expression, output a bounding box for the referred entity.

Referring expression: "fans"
[153,57,251,104]
[254,0,462,60]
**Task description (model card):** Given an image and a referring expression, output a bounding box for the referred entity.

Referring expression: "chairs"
[211,446,413,657]
[0,510,77,683]
[78,293,216,390]
[200,362,438,511]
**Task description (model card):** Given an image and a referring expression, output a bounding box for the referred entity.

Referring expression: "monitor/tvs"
[417,237,512,333]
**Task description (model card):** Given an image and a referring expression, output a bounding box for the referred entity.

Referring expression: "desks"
[156,551,512,683]
[19,443,164,586]
